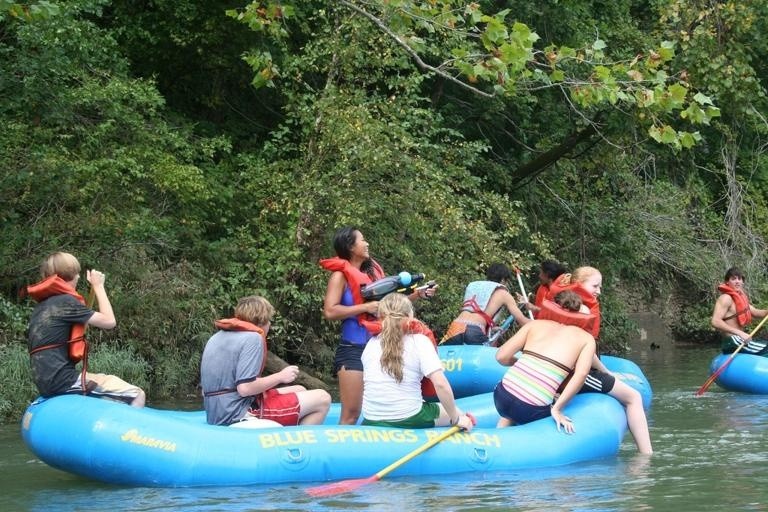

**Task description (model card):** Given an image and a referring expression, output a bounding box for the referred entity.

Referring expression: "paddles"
[696,314,768,396]
[305,425,463,501]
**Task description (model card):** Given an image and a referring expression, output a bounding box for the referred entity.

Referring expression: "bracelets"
[451,412,462,425]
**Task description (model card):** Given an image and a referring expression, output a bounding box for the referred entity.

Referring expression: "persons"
[515,257,570,316]
[710,268,767,384]
[361,291,477,431]
[321,228,442,424]
[200,295,332,425]
[543,266,655,459]
[494,288,598,434]
[441,261,534,347]
[22,250,146,411]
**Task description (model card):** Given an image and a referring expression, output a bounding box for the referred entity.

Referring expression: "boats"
[13,385,638,485]
[711,351,767,393]
[428,345,655,408]
